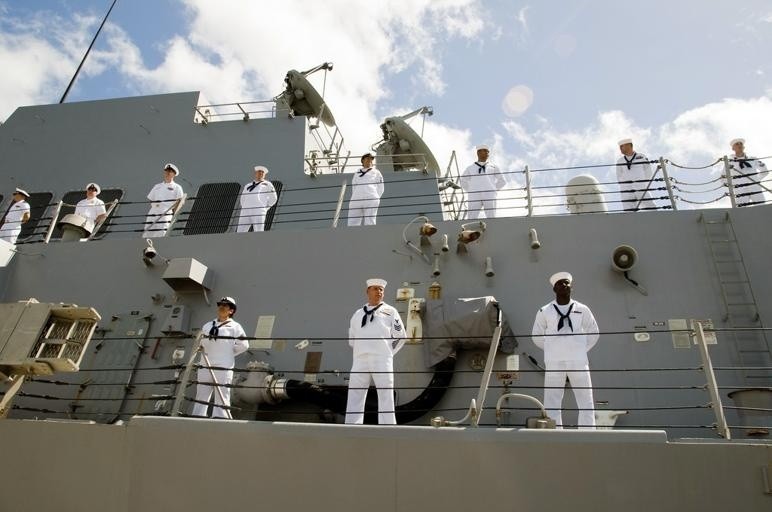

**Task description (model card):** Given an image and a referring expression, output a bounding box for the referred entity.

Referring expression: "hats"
[361,154,374,162]
[13,188,30,197]
[254,166,268,174]
[730,139,745,146]
[619,139,632,145]
[86,183,100,195]
[216,297,236,305]
[367,279,387,288]
[477,145,489,151]
[550,272,572,287]
[165,164,179,176]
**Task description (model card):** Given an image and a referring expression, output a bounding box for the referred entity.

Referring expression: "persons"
[192,297,249,419]
[75,183,106,235]
[348,153,384,226]
[0,187,30,245]
[345,279,407,425]
[616,140,656,210]
[531,271,599,429]
[721,138,768,206]
[143,164,183,237]
[237,166,278,232]
[462,145,505,218]
